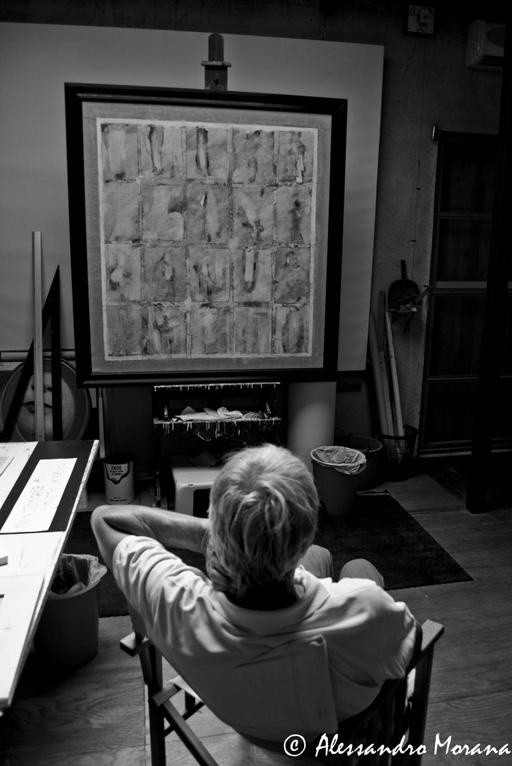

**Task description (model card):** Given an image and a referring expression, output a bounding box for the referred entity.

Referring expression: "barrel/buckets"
[102,458,134,505]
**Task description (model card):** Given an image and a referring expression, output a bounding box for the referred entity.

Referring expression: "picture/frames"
[63,79,349,385]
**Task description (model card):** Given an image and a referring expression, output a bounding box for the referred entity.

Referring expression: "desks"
[0,437,100,709]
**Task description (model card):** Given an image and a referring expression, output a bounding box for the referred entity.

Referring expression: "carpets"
[61,488,475,619]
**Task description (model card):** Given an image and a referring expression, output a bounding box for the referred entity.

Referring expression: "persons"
[90,440,417,756]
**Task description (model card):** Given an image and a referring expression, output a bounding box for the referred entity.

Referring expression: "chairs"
[119,589,446,766]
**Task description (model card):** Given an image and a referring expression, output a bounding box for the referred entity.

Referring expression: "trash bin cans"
[311,446,366,518]
[34,554,107,668]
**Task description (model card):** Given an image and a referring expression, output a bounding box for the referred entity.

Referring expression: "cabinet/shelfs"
[397,127,512,460]
